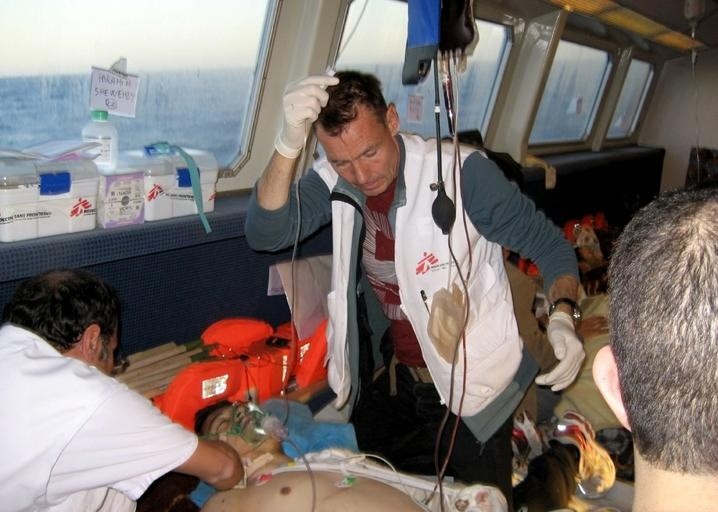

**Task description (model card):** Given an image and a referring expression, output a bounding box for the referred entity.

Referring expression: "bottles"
[81,110,118,176]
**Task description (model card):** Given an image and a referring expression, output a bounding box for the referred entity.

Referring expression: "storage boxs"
[122,148,219,223]
[0,157,100,244]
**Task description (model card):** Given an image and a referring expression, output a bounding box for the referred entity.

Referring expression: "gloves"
[274,75,340,159]
[534,312,585,391]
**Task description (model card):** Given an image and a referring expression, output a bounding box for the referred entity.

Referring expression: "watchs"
[548,296,583,326]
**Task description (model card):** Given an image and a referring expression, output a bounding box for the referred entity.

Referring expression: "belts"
[389,358,434,395]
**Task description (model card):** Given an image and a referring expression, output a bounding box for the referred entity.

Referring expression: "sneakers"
[548,412,616,500]
[511,410,545,465]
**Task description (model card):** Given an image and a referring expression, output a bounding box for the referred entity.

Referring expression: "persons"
[242,70,617,511]
[591,185,718,512]
[0,266,245,512]
[188,399,508,512]
